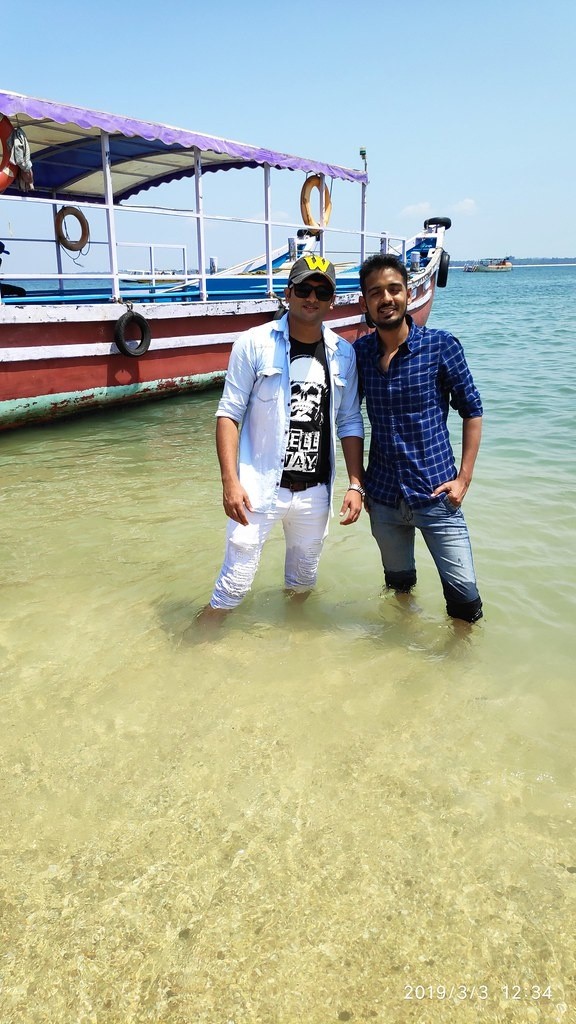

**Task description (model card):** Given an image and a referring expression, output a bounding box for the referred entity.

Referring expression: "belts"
[278,476,323,492]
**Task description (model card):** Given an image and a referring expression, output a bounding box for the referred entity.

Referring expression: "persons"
[351,254,483,625]
[0,240,12,266]
[209,254,363,613]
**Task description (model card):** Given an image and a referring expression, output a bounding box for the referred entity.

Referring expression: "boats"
[0,92,452,435]
[472,258,512,271]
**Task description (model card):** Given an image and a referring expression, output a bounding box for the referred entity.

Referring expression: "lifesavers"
[437,252,450,288]
[0,113,19,194]
[299,175,332,236]
[423,216,452,230]
[112,311,152,358]
[54,206,90,252]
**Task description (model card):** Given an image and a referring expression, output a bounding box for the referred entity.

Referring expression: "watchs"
[345,485,366,495]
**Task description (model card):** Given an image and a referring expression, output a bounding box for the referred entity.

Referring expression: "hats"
[288,254,336,289]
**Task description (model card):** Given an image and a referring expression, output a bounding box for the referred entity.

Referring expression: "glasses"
[289,280,335,302]
